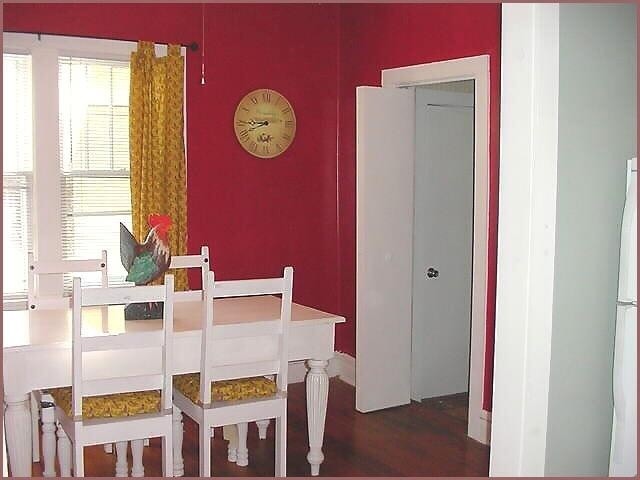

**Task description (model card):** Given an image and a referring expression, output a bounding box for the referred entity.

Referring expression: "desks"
[2,295,345,478]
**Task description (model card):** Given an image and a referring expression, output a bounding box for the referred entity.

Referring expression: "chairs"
[52,273,174,478]
[28,248,108,477]
[170,246,214,438]
[172,266,293,478]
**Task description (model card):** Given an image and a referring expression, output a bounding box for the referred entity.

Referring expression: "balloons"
[232,86,299,162]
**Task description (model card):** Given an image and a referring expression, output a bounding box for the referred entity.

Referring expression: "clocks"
[232,86,299,162]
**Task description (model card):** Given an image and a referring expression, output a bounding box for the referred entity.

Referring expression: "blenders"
[118,213,176,312]
